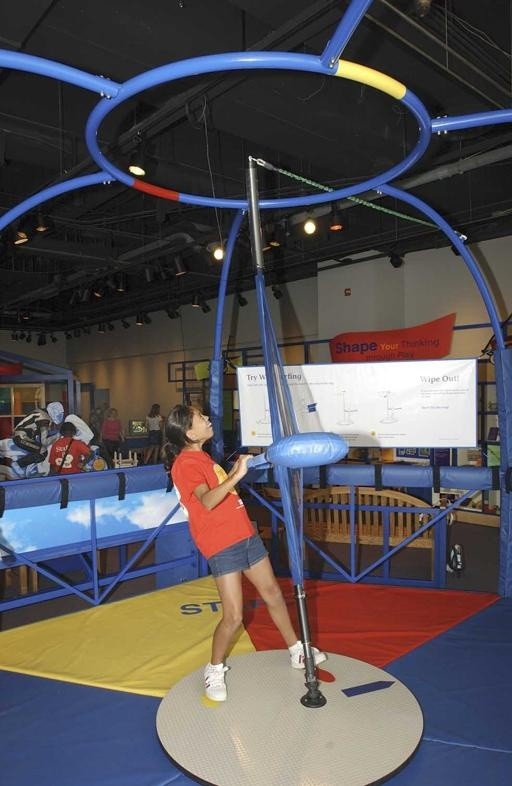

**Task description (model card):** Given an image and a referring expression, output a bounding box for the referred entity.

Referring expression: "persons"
[160,404,329,704]
[8,400,64,479]
[48,422,94,476]
[89,401,126,469]
[143,403,163,465]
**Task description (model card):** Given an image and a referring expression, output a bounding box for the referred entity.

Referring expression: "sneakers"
[203,664,227,702]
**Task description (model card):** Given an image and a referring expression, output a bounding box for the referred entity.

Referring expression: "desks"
[125,437,150,462]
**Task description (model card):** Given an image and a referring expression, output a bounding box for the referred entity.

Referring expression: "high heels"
[289,647,326,670]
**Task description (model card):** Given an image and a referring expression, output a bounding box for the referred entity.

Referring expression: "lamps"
[13,208,47,244]
[94,280,107,297]
[192,295,211,313]
[214,246,226,260]
[116,273,127,292]
[10,311,151,343]
[142,266,153,282]
[271,284,282,299]
[237,295,247,307]
[128,151,148,175]
[174,256,185,276]
[168,311,180,319]
[262,224,283,251]
[303,216,317,235]
[329,213,343,231]
